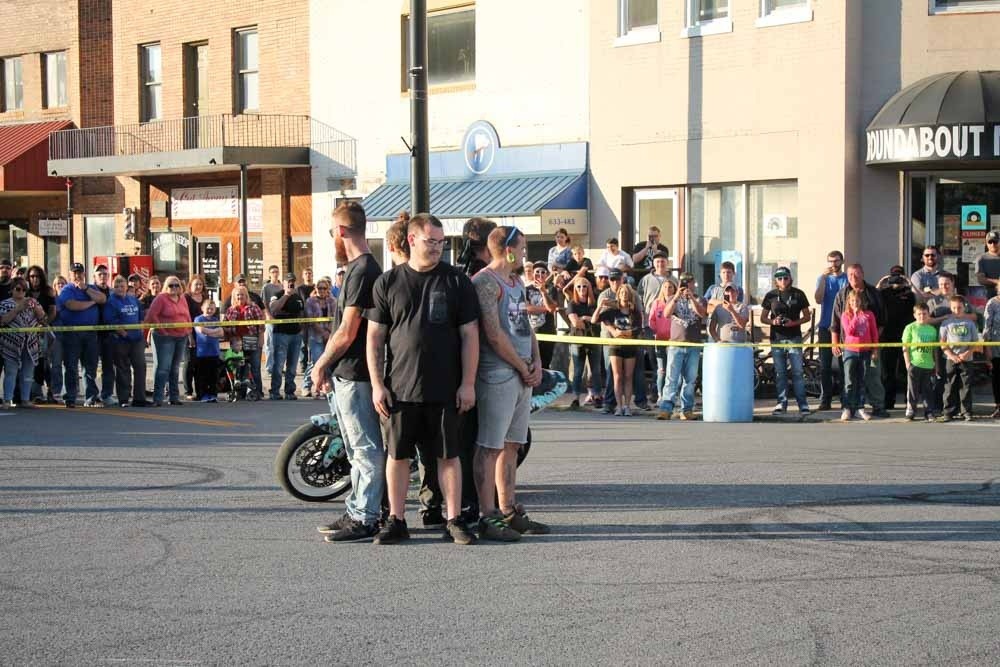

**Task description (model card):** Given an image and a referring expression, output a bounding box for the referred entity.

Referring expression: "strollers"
[217,347,262,403]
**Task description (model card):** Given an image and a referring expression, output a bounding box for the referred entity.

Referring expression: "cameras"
[779,316,787,325]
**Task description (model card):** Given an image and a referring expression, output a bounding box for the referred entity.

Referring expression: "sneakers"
[318,501,551,546]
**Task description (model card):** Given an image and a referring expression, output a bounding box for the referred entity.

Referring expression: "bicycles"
[751,348,835,397]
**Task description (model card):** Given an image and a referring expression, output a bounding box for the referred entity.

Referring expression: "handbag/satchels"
[243,336,259,350]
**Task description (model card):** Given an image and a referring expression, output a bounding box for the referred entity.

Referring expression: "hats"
[774,267,790,277]
[533,261,548,270]
[725,282,737,291]
[70,263,83,272]
[128,274,139,281]
[653,250,667,259]
[95,264,107,271]
[235,274,246,282]
[609,268,622,279]
[986,232,999,240]
[284,273,296,280]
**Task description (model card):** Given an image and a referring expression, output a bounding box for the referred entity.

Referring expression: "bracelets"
[773,319,775,325]
[13,309,19,315]
[84,284,90,291]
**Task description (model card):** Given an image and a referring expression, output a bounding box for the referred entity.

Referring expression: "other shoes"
[570,393,1000,423]
[0,388,327,411]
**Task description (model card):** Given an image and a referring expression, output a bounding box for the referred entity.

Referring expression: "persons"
[975,232,1000,299]
[524,226,750,421]
[760,267,811,415]
[366,213,480,545]
[817,246,998,422]
[0,259,346,412]
[310,198,545,543]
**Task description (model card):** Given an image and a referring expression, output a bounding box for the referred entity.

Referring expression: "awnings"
[864,68,1000,166]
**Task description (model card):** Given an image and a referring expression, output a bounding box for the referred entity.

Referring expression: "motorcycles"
[272,366,569,503]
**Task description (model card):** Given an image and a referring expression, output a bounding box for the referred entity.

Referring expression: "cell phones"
[604,299,619,307]
[724,295,729,304]
[535,274,540,283]
[888,276,904,284]
[290,284,294,289]
[681,279,688,289]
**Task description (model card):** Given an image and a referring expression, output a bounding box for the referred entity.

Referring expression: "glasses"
[988,240,998,244]
[505,226,522,245]
[925,254,936,257]
[536,270,546,274]
[30,275,39,278]
[168,284,180,288]
[611,277,620,281]
[14,288,26,291]
[330,226,347,238]
[416,236,448,248]
[0,265,10,270]
[778,277,788,281]
[318,287,327,291]
[577,284,586,289]
